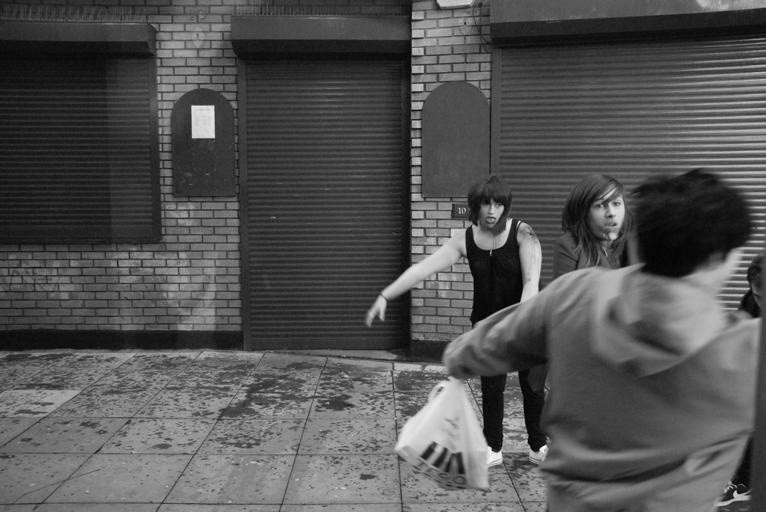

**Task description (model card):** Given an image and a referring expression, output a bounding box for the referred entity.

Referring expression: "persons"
[443,166,766,512]
[552,173,642,280]
[713,250,766,508]
[363,173,551,466]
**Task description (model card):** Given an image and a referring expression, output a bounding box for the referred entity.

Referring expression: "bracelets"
[378,292,390,302]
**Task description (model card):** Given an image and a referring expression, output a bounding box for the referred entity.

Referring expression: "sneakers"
[487,448,503,467]
[713,480,752,507]
[528,444,549,466]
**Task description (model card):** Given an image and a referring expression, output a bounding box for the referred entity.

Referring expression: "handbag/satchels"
[394,377,492,492]
